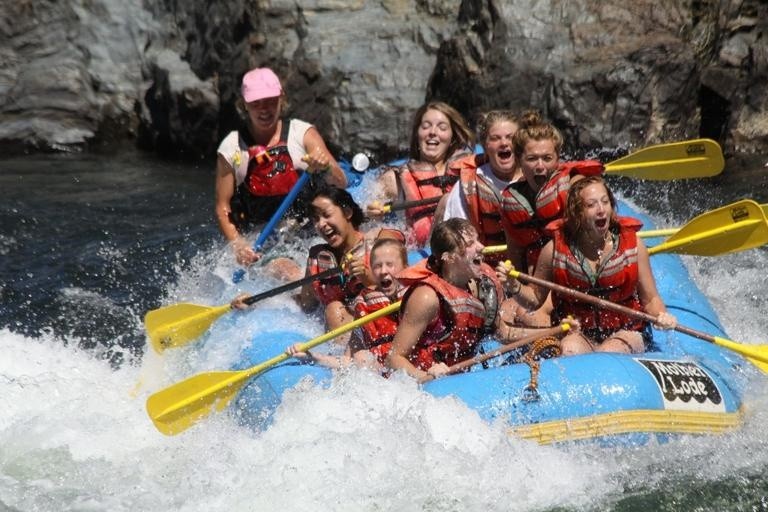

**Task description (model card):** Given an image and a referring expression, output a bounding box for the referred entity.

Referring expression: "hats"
[241,67,282,103]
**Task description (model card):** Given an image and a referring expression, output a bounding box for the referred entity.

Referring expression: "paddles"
[372,139,725,214]
[647,199,767,256]
[504,260,767,376]
[144,253,353,355]
[128,154,317,398]
[147,301,402,435]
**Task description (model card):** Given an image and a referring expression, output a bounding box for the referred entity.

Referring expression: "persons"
[285,239,415,379]
[366,100,477,248]
[498,110,605,327]
[427,109,524,270]
[381,218,581,384]
[215,68,349,284]
[231,184,373,346]
[496,176,677,355]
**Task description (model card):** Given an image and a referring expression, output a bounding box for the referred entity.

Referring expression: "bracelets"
[511,283,522,296]
[304,360,316,366]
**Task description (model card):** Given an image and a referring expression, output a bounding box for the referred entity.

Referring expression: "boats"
[195,143,764,452]
